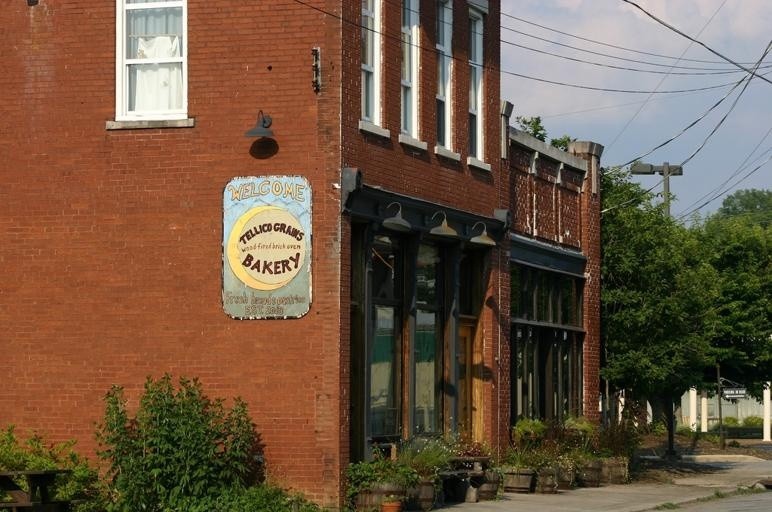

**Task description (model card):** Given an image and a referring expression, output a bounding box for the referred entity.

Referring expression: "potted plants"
[503,413,630,493]
[338,416,500,511]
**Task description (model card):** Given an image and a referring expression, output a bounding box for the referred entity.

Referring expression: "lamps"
[243,109,274,138]
[381,201,498,248]
